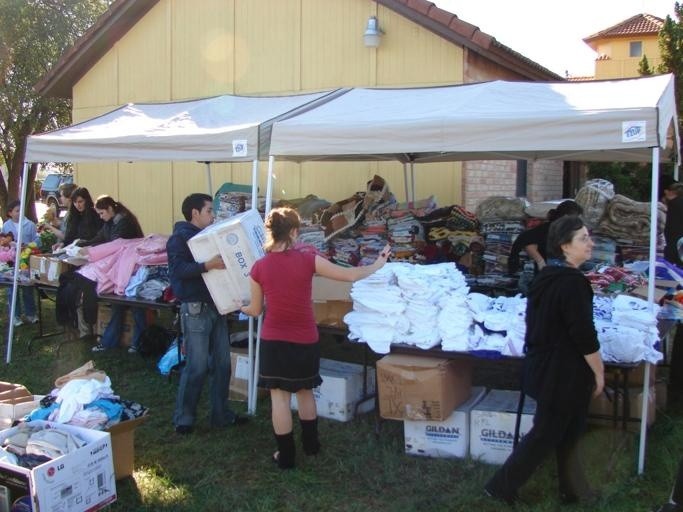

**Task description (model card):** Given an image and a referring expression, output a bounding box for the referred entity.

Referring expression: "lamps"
[361,13,383,50]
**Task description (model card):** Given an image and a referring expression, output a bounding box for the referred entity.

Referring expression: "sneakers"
[175,423,193,434]
[92,343,120,352]
[210,414,246,429]
[13,316,24,326]
[560,487,602,507]
[25,315,39,322]
[486,479,518,504]
[128,344,148,353]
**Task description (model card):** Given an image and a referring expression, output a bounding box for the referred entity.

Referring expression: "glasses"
[576,236,591,242]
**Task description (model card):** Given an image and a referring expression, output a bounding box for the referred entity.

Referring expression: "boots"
[300,417,319,454]
[274,432,295,468]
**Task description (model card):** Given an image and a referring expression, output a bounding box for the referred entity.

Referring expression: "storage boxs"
[0,381,29,401]
[586,363,655,422]
[107,406,150,482]
[0,395,48,426]
[225,345,272,406]
[374,352,481,423]
[310,274,354,331]
[184,207,275,316]
[289,356,377,425]
[29,252,70,287]
[94,300,136,348]
[402,383,489,463]
[468,388,537,465]
[0,421,117,512]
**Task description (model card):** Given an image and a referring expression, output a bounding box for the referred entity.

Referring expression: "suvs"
[39,173,74,211]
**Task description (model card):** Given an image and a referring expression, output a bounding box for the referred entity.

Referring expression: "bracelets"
[239,304,249,310]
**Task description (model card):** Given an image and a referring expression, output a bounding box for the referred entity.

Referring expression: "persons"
[0,200,41,326]
[230,206,393,468]
[660,177,683,269]
[507,198,585,282]
[43,183,78,331]
[76,194,145,354]
[482,214,606,509]
[55,186,104,340]
[165,192,251,436]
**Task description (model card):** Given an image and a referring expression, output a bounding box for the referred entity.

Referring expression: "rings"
[382,253,385,257]
